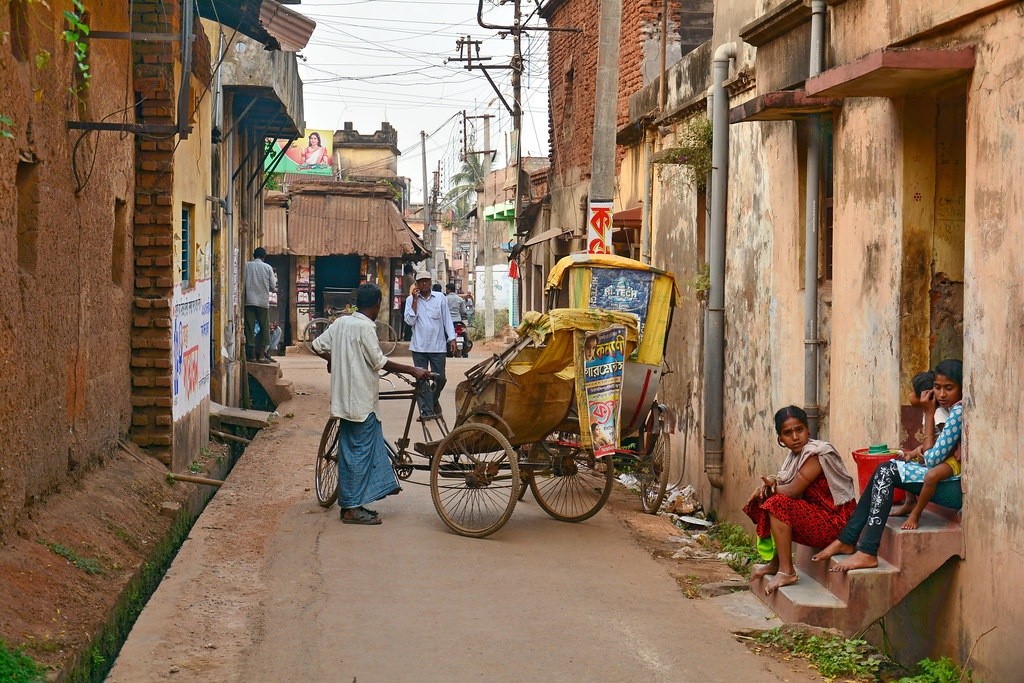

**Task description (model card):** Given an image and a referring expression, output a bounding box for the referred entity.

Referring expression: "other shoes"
[463,355,468,358]
[257,357,270,363]
[248,357,257,362]
[446,353,453,358]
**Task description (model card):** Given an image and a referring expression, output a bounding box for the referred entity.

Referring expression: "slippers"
[339,508,379,521]
[342,510,383,525]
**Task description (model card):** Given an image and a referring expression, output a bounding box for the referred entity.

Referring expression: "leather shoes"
[416,412,431,422]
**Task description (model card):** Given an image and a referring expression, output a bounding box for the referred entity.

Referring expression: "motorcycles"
[445,320,474,359]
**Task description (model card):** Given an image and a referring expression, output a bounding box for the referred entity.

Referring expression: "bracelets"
[923,436,934,439]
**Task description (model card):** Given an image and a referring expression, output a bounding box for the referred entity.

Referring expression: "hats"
[414,270,432,280]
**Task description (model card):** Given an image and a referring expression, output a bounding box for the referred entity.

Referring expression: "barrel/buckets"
[851,448,906,504]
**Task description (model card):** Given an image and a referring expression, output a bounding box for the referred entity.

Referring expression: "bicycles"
[303,305,398,358]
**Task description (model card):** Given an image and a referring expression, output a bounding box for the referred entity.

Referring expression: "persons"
[253,318,282,362]
[585,335,599,361]
[241,247,276,363]
[406,271,458,421]
[313,283,430,525]
[402,265,415,341]
[301,131,328,166]
[591,423,610,450]
[811,358,963,571]
[433,284,442,292]
[749,405,856,597]
[463,291,474,307]
[446,284,466,322]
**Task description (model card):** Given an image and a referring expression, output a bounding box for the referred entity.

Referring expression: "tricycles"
[314,252,683,537]
